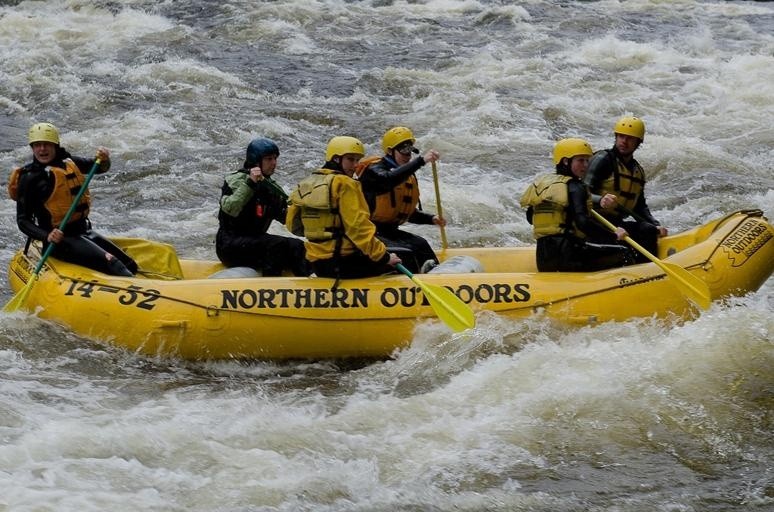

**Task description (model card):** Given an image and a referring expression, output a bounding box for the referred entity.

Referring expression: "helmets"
[27,122,60,146]
[552,138,593,165]
[613,116,645,144]
[325,135,365,162]
[380,126,416,155]
[245,137,280,165]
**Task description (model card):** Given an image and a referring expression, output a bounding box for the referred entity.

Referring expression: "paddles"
[393,260,475,333]
[591,208,712,311]
[3,157,101,312]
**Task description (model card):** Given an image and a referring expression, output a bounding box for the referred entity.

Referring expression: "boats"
[9,206,773,367]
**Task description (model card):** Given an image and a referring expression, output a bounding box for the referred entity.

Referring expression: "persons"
[586,115,668,259]
[213,137,310,278]
[520,136,635,273]
[8,121,140,278]
[284,132,405,278]
[355,125,446,273]
